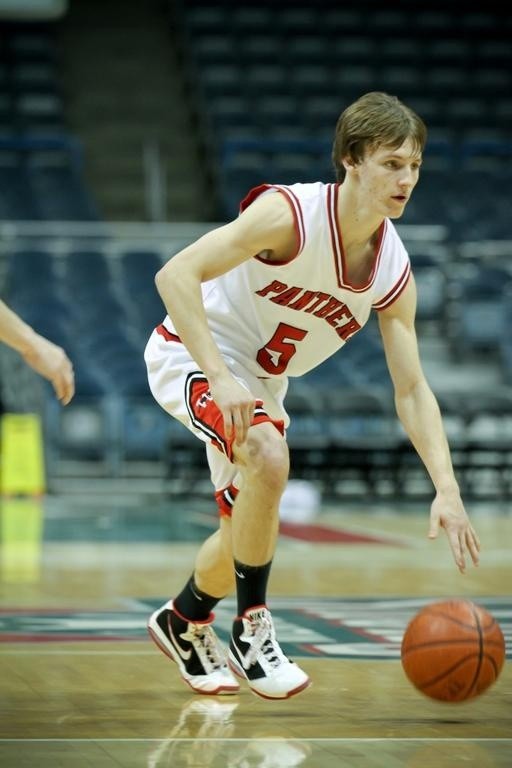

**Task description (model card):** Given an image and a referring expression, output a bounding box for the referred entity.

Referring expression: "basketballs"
[401,602,504,700]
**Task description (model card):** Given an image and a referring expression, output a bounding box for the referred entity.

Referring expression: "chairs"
[2,16,101,220]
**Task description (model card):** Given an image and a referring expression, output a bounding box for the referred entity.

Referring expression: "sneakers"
[148,599,239,694]
[226,605,310,699]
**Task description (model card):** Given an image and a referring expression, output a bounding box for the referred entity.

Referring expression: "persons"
[140,90,480,698]
[0,296,77,404]
